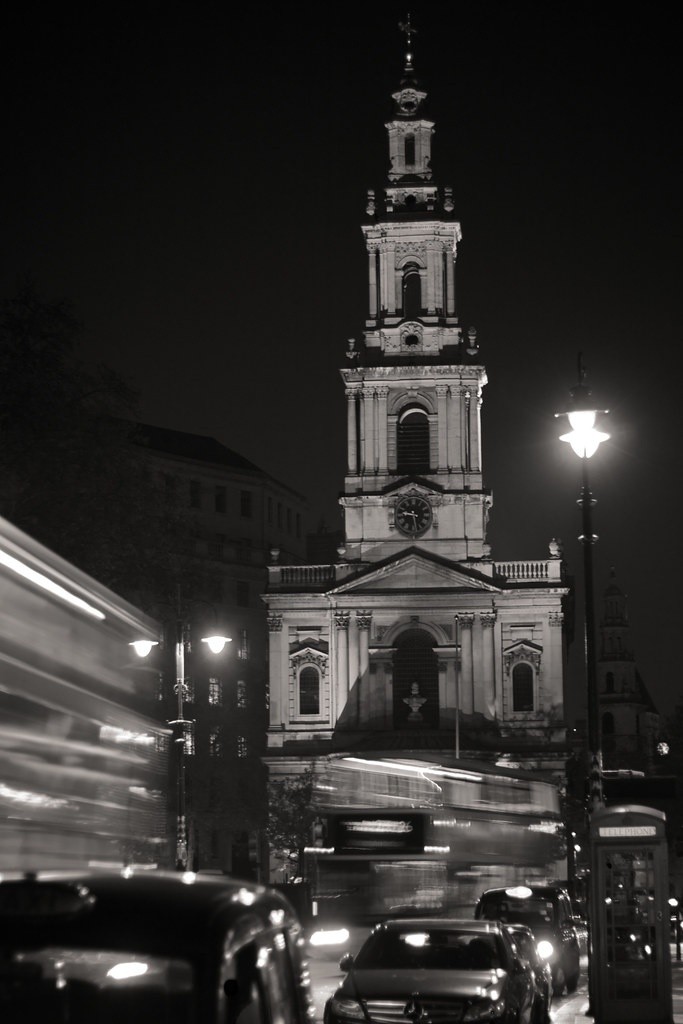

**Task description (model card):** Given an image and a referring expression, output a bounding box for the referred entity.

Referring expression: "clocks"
[394,497,434,538]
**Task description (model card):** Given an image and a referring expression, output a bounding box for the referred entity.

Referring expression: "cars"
[323,916,554,1024]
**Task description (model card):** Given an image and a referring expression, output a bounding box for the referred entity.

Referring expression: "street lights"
[124,596,234,870]
[548,349,613,814]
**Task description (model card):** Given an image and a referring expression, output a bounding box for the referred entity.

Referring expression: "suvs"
[472,884,581,996]
[0,864,312,1024]
